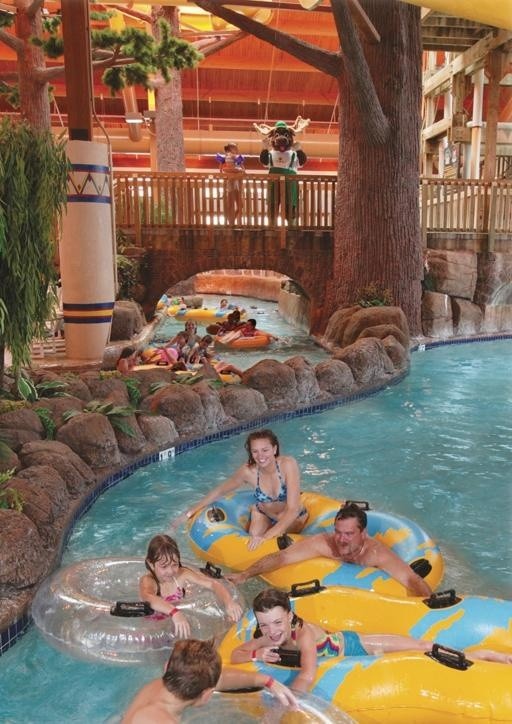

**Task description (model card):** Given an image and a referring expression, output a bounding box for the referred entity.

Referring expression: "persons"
[116,294,278,378]
[185,429,309,552]
[216,144,245,226]
[119,639,299,724]
[138,535,243,640]
[223,503,434,597]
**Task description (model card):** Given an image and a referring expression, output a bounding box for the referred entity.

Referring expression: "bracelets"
[251,649,256,658]
[265,676,273,688]
[169,607,180,616]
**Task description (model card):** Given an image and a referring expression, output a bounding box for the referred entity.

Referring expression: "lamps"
[124,111,155,135]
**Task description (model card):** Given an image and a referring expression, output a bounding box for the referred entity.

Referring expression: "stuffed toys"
[253,115,311,226]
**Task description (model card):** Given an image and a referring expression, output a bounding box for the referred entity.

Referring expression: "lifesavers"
[32,557,249,668]
[187,486,444,600]
[157,294,167,309]
[175,304,248,323]
[141,341,237,382]
[214,332,269,349]
[104,684,359,724]
